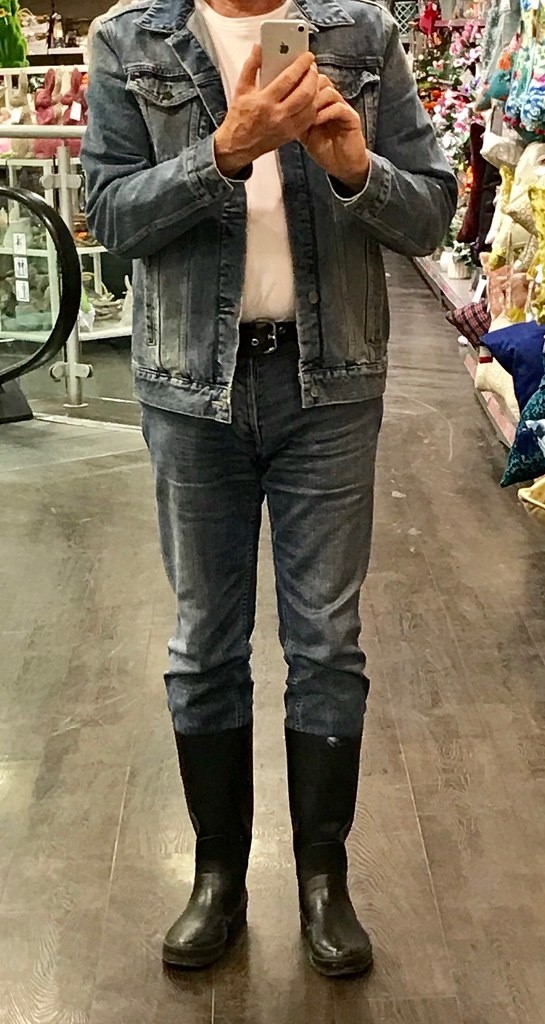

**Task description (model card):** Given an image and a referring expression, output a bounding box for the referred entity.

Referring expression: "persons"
[81,0,458,977]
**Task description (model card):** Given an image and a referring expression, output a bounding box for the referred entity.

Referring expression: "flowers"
[416,18,485,197]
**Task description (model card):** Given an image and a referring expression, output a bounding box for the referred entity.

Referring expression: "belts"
[236,319,295,354]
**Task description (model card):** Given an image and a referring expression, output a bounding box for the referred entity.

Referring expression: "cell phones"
[258,19,309,90]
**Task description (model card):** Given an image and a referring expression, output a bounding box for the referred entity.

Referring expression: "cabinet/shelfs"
[1,64,133,344]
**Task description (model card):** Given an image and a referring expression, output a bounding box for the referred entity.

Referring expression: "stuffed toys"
[0,69,89,161]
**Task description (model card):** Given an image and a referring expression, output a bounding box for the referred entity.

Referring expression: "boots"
[163,721,254,967]
[285,728,374,977]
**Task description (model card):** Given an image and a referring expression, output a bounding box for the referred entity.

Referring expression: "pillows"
[445,174,545,524]
